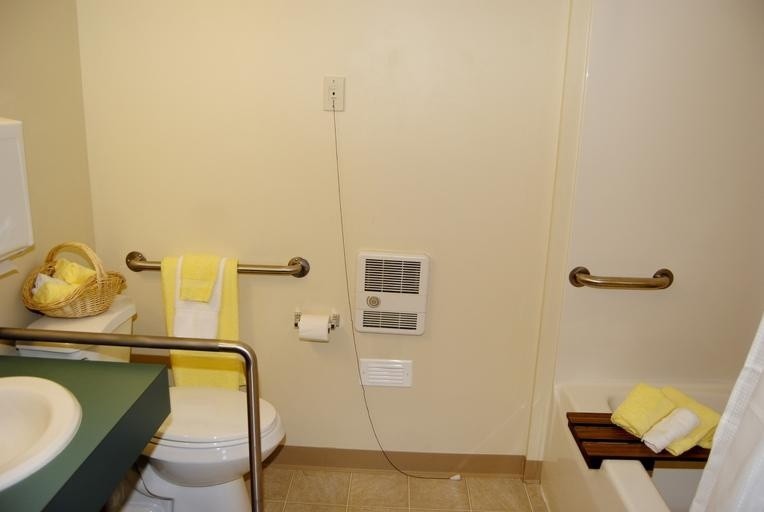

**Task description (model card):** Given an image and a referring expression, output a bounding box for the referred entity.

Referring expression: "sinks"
[0,376,82,492]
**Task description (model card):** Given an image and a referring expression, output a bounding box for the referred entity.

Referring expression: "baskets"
[21,241,126,319]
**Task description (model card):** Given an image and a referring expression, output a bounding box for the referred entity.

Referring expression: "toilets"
[16,294,284,512]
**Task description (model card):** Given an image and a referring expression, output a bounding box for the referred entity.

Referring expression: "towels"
[31,258,98,304]
[611,384,723,456]
[162,253,247,393]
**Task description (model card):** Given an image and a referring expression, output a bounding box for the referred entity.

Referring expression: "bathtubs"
[562,384,731,512]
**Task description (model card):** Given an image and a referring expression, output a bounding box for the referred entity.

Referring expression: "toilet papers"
[299,315,331,342]
